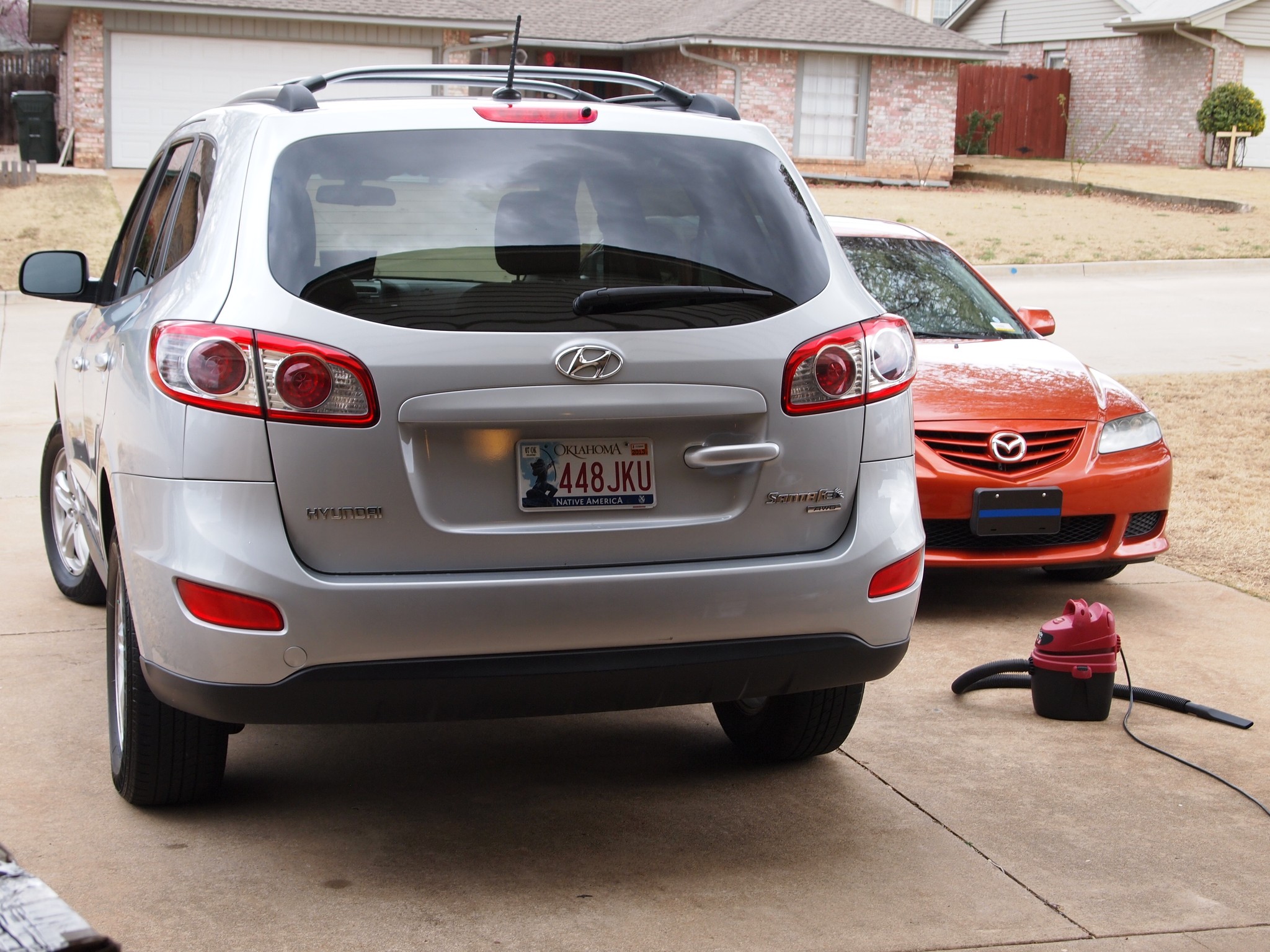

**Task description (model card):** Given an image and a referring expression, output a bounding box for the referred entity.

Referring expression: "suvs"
[19,65,927,808]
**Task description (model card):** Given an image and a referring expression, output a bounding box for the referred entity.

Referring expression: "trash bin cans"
[10,90,59,163]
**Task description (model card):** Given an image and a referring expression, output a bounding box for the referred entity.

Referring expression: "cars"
[583,216,1174,585]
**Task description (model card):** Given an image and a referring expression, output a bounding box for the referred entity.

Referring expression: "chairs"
[454,190,582,315]
